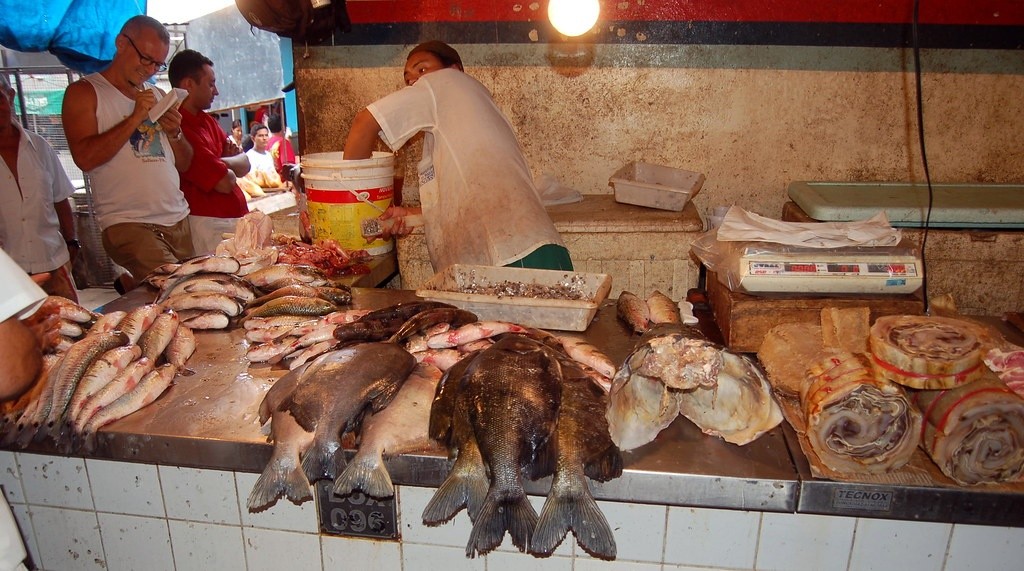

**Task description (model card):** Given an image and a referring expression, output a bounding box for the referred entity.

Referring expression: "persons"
[61,14,198,295]
[168,49,251,255]
[228,119,259,152]
[343,36,574,275]
[0,71,81,304]
[0,246,48,571]
[265,113,296,182]
[246,123,280,179]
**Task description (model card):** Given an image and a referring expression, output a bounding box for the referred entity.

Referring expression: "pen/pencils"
[128,80,143,92]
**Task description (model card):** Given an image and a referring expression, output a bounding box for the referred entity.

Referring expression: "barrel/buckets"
[302,151,395,257]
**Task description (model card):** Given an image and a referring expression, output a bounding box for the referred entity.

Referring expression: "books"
[148,87,189,124]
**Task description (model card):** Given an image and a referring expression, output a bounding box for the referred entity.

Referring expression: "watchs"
[169,128,183,142]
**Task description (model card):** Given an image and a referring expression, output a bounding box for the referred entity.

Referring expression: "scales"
[716,235,924,297]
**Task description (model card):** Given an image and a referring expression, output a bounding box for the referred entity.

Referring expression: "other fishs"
[645,288,683,327]
[244,300,625,562]
[0,302,199,460]
[139,254,355,328]
[615,291,650,338]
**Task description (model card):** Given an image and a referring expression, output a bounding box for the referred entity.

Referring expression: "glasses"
[122,33,168,73]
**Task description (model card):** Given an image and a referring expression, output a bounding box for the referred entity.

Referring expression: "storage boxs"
[395,194,703,302]
[610,161,704,211]
[416,263,611,332]
